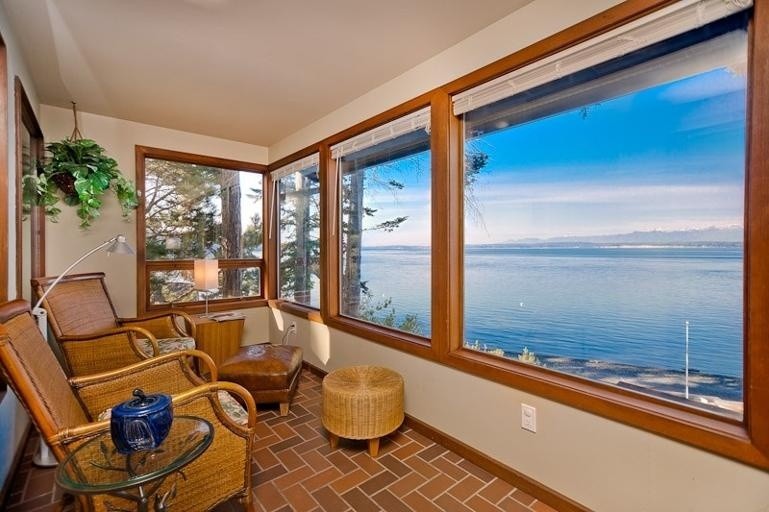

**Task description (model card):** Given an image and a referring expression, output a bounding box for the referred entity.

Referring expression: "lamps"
[194,256,218,319]
[32,234,136,342]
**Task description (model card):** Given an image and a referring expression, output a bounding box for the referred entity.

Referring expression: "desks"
[52,414,214,512]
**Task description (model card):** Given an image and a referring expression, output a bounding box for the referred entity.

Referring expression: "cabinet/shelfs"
[185,311,246,377]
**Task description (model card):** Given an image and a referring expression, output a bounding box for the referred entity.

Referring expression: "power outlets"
[289,320,296,335]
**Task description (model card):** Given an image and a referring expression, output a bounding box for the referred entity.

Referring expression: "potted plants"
[21,101,141,231]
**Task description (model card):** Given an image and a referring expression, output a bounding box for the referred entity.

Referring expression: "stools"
[322,365,404,456]
[207,344,303,416]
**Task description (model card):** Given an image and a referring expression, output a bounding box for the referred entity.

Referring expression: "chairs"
[1,299,257,512]
[30,272,196,377]
